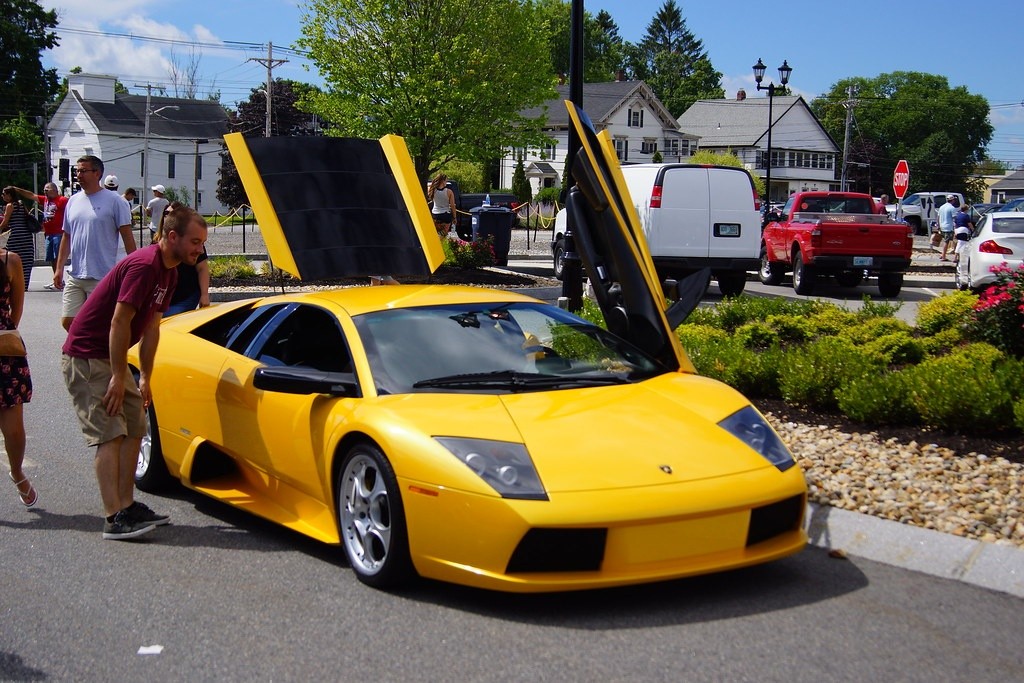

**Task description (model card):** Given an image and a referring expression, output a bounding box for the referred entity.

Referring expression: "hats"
[152,184,166,194]
[947,195,955,200]
[104,173,119,186]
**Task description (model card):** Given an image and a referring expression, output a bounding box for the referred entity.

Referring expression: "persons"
[953,204,974,262]
[14,182,68,291]
[0,246,39,507]
[145,184,169,241]
[152,201,210,318]
[57,178,70,196]
[429,173,457,236]
[875,194,889,214]
[61,206,208,540]
[937,195,958,261]
[0,186,34,291]
[53,155,137,333]
[104,174,119,195]
[122,187,137,220]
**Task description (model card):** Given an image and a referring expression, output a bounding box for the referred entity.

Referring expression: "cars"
[968,197,1024,228]
[763,201,787,215]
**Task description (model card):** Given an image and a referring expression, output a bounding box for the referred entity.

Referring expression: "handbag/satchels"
[21,201,42,233]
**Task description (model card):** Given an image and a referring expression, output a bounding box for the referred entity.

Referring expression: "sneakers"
[123,500,171,524]
[103,511,156,538]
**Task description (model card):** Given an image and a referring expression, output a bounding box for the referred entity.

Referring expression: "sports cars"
[121,95,812,598]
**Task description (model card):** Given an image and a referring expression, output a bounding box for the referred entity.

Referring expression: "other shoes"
[44,280,65,291]
[952,259,959,262]
[940,258,947,260]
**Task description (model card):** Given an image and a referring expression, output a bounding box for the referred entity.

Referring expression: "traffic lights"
[71,167,79,182]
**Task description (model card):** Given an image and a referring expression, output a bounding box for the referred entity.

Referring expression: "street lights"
[143,104,180,226]
[753,57,793,211]
[257,88,272,137]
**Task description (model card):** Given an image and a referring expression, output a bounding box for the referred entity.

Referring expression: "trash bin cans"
[469,207,516,266]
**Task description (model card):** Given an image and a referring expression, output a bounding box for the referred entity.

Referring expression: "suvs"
[954,211,1024,290]
[885,192,965,235]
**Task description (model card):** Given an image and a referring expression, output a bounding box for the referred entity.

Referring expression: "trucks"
[551,161,762,299]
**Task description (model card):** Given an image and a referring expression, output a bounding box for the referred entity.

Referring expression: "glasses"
[74,169,95,175]
[164,211,170,217]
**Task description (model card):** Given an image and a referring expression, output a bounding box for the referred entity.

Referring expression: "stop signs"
[893,160,909,198]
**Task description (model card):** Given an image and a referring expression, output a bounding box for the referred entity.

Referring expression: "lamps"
[717,123,721,129]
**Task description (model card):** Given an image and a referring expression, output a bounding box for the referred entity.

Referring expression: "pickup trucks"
[758,190,915,297]
[427,179,530,227]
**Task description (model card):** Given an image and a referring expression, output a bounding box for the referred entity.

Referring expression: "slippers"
[9,471,38,506]
